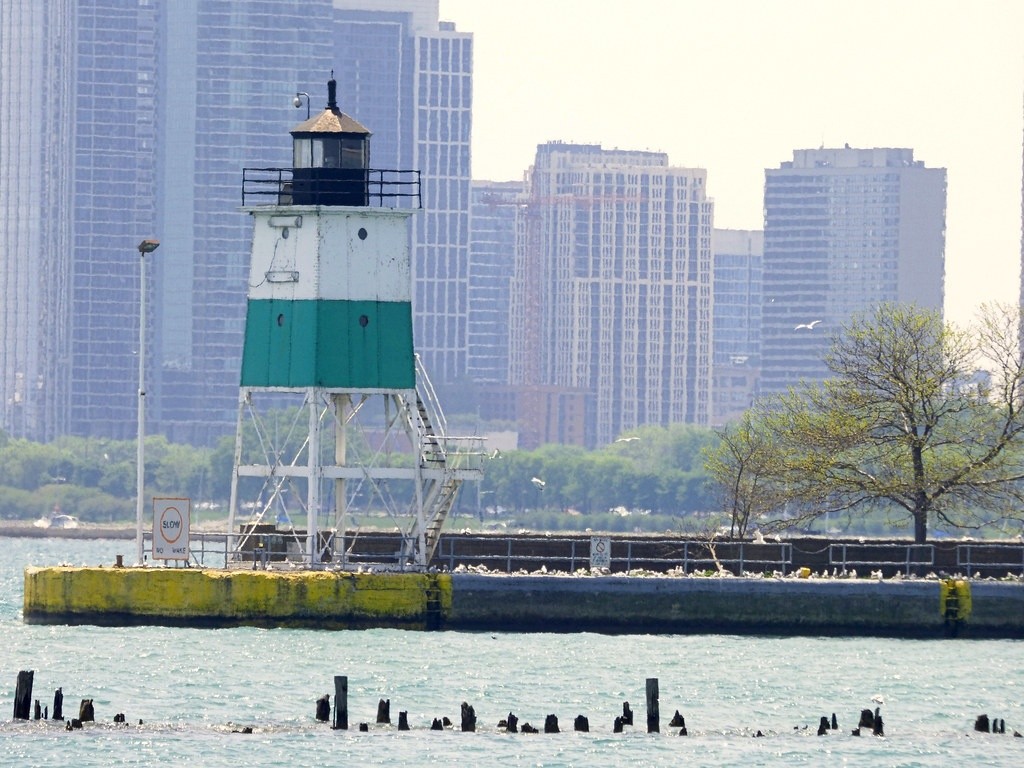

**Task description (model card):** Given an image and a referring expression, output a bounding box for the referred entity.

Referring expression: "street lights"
[132,237,161,567]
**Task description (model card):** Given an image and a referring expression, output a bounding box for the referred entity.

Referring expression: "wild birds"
[531,477,545,491]
[794,320,824,330]
[261,558,1024,586]
[488,448,504,461]
[615,437,640,443]
